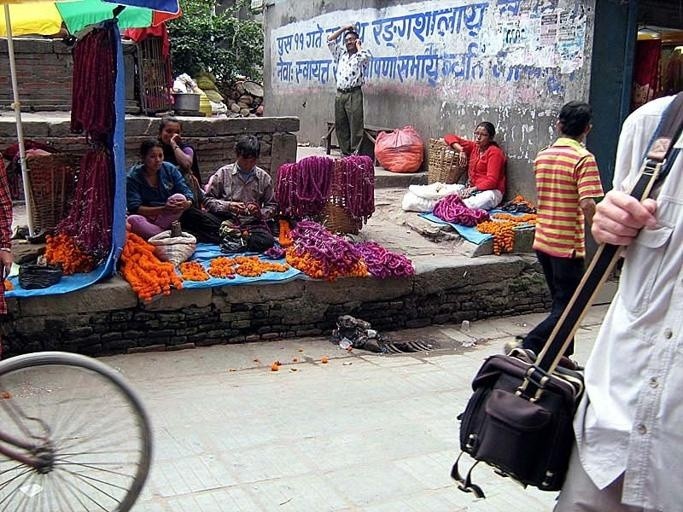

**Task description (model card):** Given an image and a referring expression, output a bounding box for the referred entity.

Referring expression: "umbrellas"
[0,0,182,237]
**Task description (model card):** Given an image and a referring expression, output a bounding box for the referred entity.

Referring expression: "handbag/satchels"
[443,345,587,503]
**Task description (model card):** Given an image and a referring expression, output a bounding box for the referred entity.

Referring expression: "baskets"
[426,136,467,184]
[24,153,85,235]
[312,157,369,234]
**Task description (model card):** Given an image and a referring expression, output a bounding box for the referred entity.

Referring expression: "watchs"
[172,145,180,150]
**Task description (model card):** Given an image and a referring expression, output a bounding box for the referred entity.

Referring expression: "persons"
[158,118,202,210]
[0,152,13,399]
[443,121,507,211]
[326,24,369,158]
[552,95,683,512]
[125,139,196,241]
[503,100,605,372]
[180,135,279,253]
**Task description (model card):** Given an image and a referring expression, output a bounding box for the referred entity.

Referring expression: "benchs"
[322,121,394,167]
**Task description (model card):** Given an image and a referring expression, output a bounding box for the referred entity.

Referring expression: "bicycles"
[0,351,152,512]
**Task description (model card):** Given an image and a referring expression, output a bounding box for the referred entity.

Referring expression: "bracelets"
[0,247,11,253]
